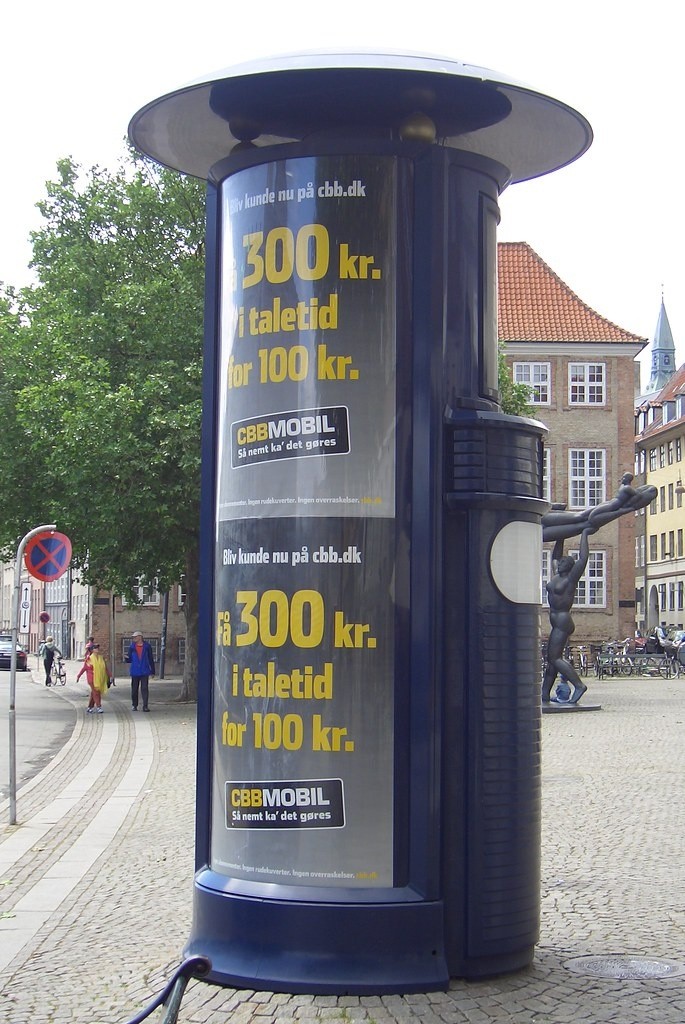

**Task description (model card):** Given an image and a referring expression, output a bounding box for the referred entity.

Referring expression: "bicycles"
[50,654,67,685]
[541,637,685,680]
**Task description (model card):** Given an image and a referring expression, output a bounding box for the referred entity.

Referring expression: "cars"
[635,625,685,666]
[0,635,29,671]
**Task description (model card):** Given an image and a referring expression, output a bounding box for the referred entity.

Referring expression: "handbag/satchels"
[45,646,54,659]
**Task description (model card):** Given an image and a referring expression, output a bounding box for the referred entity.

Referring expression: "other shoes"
[143,707,149,712]
[132,705,137,711]
[86,707,94,713]
[96,707,103,713]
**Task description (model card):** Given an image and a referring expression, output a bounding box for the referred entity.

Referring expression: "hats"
[89,645,99,649]
[131,631,143,637]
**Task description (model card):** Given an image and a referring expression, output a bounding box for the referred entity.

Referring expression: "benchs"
[596,652,671,679]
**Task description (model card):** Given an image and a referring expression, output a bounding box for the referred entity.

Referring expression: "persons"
[124,631,156,712]
[540,471,659,705]
[41,636,61,687]
[75,636,112,714]
[550,674,571,703]
